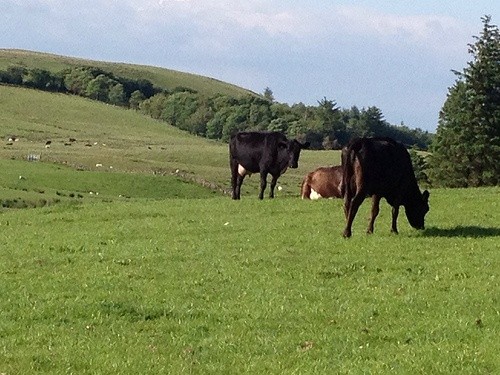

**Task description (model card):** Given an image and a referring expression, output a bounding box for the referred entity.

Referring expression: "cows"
[228,131,311,202]
[301,164,374,202]
[340,137,430,239]
